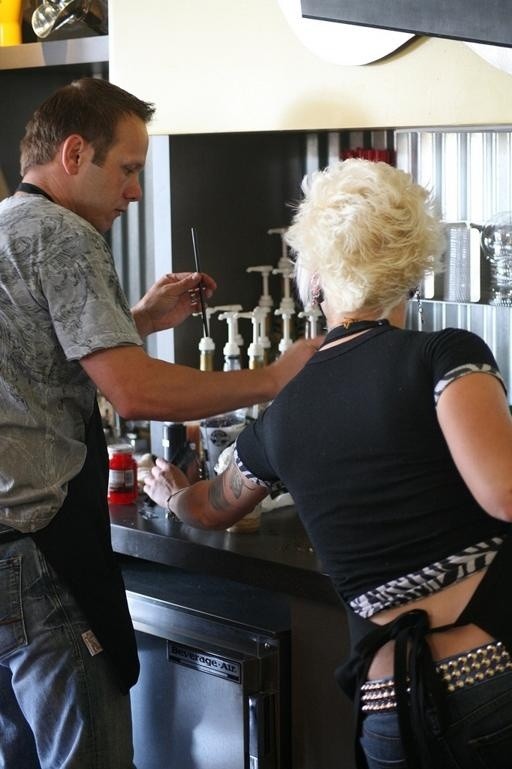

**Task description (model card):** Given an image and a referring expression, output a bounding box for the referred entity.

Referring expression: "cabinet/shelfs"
[110,496,362,769]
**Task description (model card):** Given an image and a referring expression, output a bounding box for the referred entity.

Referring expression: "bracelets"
[167,488,186,515]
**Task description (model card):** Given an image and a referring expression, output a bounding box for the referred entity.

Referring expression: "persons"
[0,78,326,769]
[141,158,511,768]
